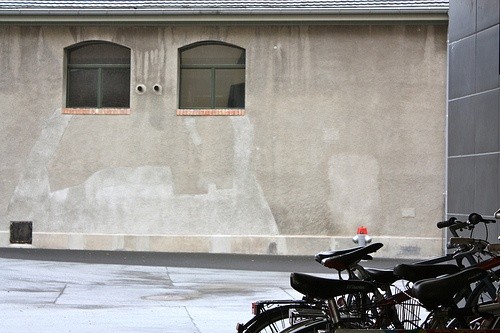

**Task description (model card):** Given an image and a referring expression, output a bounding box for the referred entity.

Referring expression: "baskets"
[367,284,423,329]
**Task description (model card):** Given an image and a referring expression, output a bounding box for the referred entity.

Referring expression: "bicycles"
[235,209,500,333]
